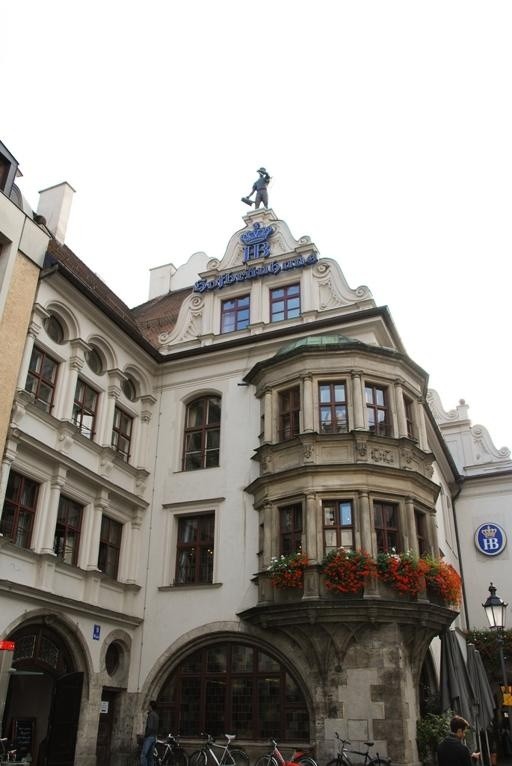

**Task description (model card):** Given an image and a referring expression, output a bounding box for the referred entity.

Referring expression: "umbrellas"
[468,640,498,765]
[441,625,479,747]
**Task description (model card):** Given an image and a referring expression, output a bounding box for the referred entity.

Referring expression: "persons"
[437,716,480,765]
[247,167,269,208]
[140,700,160,766]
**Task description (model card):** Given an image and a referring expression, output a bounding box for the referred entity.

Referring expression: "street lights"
[482,583,510,695]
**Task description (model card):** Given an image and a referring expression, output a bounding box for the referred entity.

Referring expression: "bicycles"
[189,728,249,766]
[126,734,189,765]
[326,732,391,765]
[255,737,317,766]
